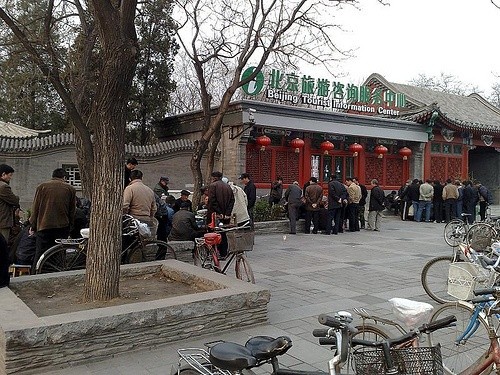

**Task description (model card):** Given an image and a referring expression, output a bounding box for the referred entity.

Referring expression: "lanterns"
[290,137,304,153]
[320,141,334,154]
[256,135,271,151]
[399,147,411,160]
[374,144,388,159]
[348,142,362,156]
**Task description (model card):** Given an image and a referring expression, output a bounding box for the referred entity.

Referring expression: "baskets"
[447,262,496,300]
[226,232,254,252]
[472,230,492,252]
[354,343,444,375]
[139,217,159,240]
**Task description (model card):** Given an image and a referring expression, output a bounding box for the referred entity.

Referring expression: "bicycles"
[34,213,178,275]
[190,219,255,286]
[173,201,500,375]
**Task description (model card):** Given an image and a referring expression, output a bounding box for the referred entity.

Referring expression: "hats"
[181,190,191,195]
[161,176,169,180]
[238,173,249,179]
[211,171,222,177]
[200,186,207,195]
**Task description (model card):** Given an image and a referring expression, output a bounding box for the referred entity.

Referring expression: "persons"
[8,198,91,265]
[0,164,19,259]
[124,157,138,189]
[238,173,256,232]
[398,178,479,224]
[221,175,251,232]
[153,176,209,262]
[285,180,302,234]
[269,176,283,208]
[475,181,494,222]
[29,168,76,276]
[207,171,235,261]
[384,190,399,216]
[303,176,368,234]
[122,168,157,263]
[366,178,385,231]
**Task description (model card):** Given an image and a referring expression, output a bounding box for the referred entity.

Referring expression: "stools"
[10,265,31,277]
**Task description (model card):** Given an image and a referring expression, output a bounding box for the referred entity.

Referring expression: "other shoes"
[288,226,379,235]
[415,218,451,223]
